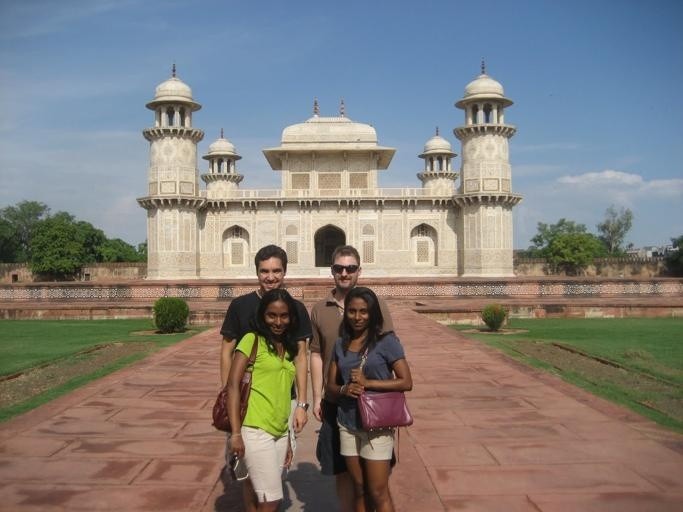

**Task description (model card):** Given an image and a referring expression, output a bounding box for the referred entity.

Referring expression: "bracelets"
[232,434,241,436]
[340,384,346,395]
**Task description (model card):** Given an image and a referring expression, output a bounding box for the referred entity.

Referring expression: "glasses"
[333,265,358,273]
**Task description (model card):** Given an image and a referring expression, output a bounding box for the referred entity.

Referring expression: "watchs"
[297,402,309,411]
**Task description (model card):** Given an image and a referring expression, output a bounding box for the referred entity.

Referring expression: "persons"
[327,286,413,512]
[220,245,312,512]
[311,246,396,512]
[227,289,296,512]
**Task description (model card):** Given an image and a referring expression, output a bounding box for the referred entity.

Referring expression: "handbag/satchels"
[357,330,413,431]
[212,332,260,431]
